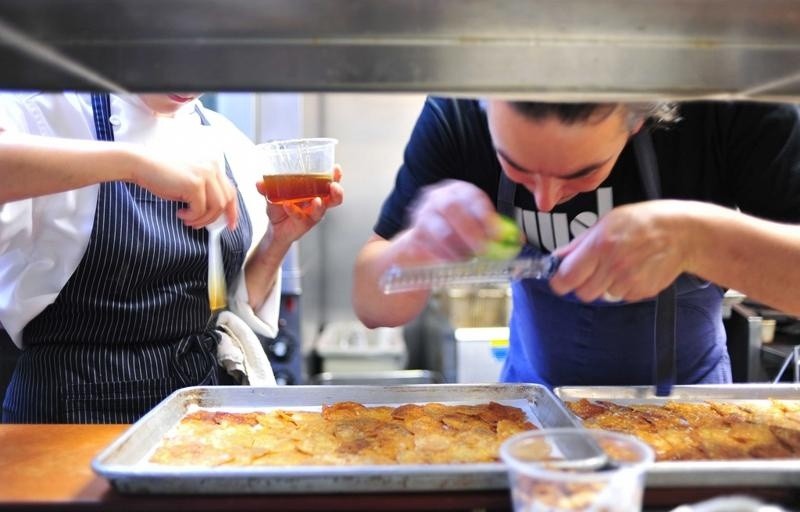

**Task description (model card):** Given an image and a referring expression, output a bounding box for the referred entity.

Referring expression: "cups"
[500,428,652,511]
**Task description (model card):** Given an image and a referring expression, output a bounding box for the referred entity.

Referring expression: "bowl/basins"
[252,137,338,202]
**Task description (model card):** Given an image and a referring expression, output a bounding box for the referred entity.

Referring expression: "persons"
[2,91,346,424]
[351,93,798,387]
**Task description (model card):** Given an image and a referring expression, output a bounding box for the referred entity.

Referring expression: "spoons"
[203,205,227,310]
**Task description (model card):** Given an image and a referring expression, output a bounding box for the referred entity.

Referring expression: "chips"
[565,397,800,461]
[149,402,551,467]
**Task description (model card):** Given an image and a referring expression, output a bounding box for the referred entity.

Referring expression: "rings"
[310,207,326,221]
[600,289,624,304]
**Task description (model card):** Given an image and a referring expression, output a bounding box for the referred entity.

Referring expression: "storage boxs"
[453,326,511,386]
[315,320,405,373]
[429,285,508,326]
